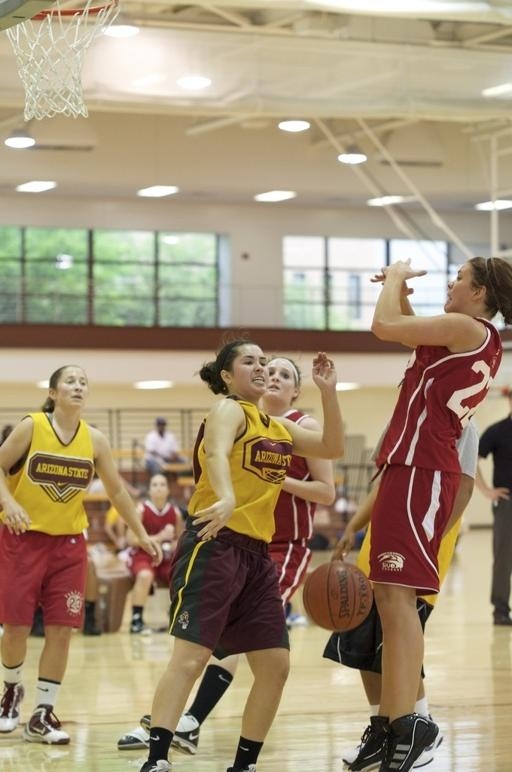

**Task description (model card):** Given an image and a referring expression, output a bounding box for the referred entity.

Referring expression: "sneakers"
[0,683,24,733]
[286,614,308,627]
[32,625,47,636]
[130,614,144,634]
[23,704,71,746]
[342,712,444,771]
[140,713,201,757]
[117,729,150,749]
[82,623,101,633]
[140,759,171,772]
[494,613,511,624]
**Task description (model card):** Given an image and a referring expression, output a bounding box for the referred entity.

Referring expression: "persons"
[0,337,345,772]
[323,256,512,770]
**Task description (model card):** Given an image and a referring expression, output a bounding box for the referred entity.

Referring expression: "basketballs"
[303,562,374,632]
